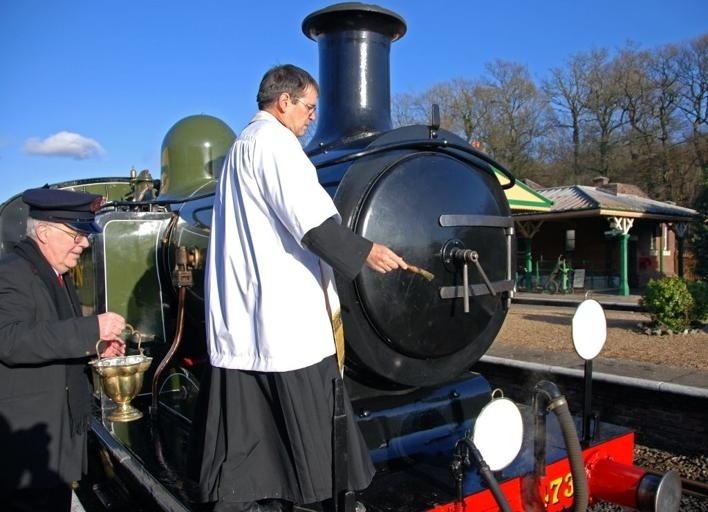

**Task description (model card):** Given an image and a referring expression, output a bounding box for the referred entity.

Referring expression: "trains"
[1,1,684,512]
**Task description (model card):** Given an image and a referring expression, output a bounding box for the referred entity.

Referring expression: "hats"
[22,189,102,233]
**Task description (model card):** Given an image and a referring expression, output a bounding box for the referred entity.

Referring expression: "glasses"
[296,99,315,116]
[46,223,89,243]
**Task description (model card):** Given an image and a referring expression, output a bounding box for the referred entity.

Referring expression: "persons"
[1,190,126,510]
[195,65,409,509]
